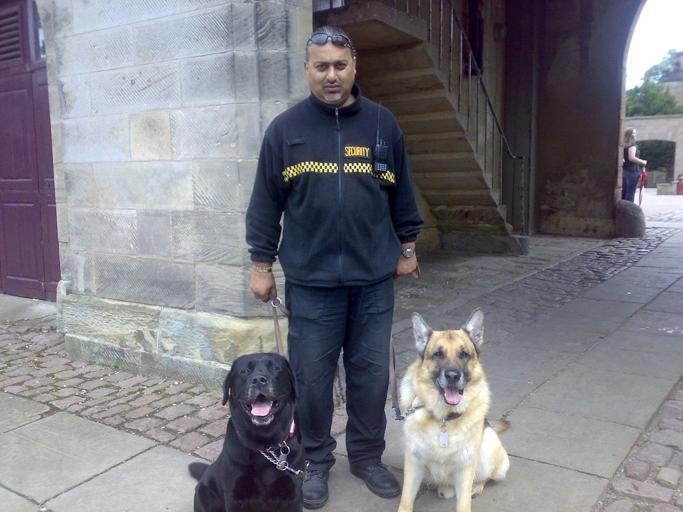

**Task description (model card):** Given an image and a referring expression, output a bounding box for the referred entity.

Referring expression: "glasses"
[309,32,351,46]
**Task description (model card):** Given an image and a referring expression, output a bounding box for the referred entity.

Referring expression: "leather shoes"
[301,467,329,509]
[349,455,400,498]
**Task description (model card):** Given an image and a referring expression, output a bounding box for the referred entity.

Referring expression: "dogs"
[396,305,511,512]
[188,351,303,512]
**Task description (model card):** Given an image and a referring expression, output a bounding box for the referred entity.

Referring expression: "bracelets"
[250,264,272,272]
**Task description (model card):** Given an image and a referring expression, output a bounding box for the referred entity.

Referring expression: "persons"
[622,128,647,202]
[246,26,418,510]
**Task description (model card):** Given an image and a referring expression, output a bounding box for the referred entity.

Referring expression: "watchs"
[402,248,415,258]
